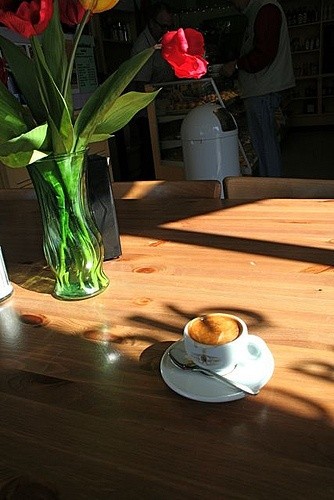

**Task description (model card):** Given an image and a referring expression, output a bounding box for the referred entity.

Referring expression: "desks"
[0,200,334,500]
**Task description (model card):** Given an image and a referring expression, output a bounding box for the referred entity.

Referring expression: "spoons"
[167,347,260,395]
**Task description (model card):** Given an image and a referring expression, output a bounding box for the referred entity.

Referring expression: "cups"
[183,313,249,377]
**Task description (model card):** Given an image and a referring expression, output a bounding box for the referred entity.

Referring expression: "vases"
[26,148,109,300]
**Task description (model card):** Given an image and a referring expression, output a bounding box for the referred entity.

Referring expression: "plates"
[160,334,274,401]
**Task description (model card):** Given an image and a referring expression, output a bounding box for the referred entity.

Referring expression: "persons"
[220,0,297,179]
[130,5,185,181]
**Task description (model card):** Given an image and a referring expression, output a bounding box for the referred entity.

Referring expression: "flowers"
[0,0,162,285]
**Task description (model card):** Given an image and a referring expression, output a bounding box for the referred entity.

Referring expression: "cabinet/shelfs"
[146,80,262,182]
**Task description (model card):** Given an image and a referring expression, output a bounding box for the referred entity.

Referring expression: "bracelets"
[235,62,238,70]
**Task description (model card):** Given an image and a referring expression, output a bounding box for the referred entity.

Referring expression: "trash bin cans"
[181,103,239,199]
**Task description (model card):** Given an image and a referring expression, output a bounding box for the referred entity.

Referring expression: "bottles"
[112,22,129,42]
[304,33,320,50]
[285,3,334,26]
[295,62,317,77]
